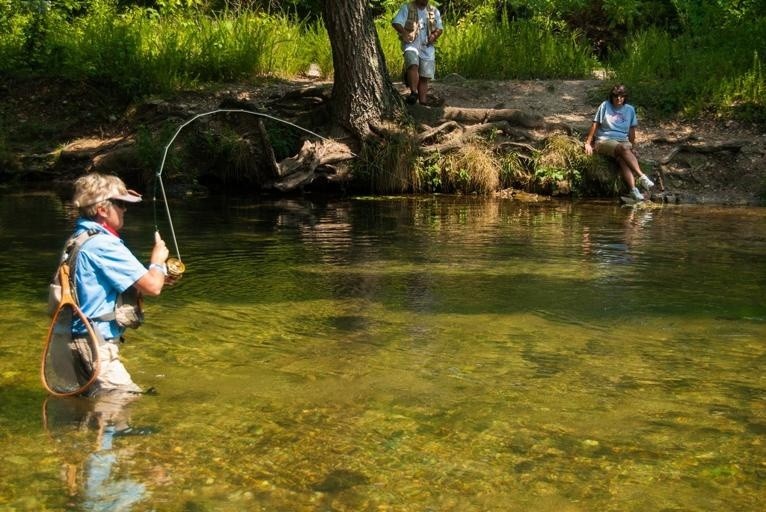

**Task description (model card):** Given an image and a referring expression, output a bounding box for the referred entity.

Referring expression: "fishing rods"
[157,109,430,287]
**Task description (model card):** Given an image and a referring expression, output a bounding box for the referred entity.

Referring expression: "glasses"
[109,199,126,209]
[612,95,627,96]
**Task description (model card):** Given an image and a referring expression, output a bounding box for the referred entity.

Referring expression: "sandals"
[112,426,161,436]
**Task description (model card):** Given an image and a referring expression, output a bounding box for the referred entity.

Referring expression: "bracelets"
[149,262,169,277]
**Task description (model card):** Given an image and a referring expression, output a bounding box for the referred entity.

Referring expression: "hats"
[72,187,142,209]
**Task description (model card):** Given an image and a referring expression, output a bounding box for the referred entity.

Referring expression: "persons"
[390,1,443,109]
[583,84,656,203]
[46,172,179,511]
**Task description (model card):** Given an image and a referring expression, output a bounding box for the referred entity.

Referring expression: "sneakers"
[639,174,654,191]
[630,187,644,202]
[408,92,418,106]
